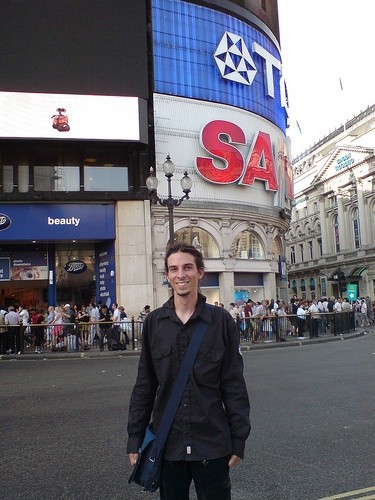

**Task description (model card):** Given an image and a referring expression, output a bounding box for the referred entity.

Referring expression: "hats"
[63,304,70,308]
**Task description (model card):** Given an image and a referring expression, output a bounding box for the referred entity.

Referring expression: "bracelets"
[64,314,66,316]
[263,315,265,318]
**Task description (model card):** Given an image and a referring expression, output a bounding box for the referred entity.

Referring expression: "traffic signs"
[347,284,357,300]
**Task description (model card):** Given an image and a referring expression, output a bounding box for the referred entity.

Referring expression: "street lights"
[146,151,193,245]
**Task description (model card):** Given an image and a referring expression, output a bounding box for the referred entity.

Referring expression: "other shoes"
[366,323,370,326]
[298,337,306,339]
[265,340,272,343]
[360,324,364,327]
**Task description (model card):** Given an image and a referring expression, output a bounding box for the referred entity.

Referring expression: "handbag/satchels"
[24,326,32,336]
[128,422,164,494]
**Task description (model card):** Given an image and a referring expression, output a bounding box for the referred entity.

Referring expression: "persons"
[0,287,375,355]
[126,244,251,500]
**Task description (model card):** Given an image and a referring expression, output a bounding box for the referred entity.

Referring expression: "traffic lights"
[339,275,347,292]
